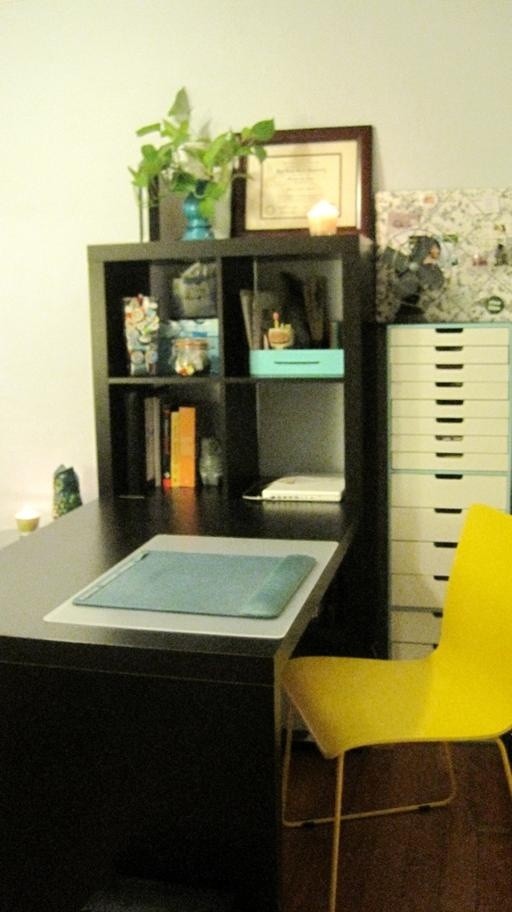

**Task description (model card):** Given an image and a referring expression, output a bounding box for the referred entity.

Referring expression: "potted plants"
[122,85,274,246]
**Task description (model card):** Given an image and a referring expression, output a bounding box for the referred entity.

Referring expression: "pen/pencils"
[271,309,286,329]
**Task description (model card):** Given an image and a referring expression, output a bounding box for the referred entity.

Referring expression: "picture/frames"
[226,122,376,242]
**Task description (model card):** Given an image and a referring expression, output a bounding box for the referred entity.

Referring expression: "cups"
[268,327,295,348]
[307,214,337,236]
[16,514,40,535]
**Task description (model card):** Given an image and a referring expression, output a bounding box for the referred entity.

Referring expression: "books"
[241,473,346,503]
[121,390,202,489]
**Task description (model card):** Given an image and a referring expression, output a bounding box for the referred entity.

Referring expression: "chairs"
[277,500,512,911]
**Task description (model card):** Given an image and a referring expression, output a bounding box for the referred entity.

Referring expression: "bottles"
[198,437,223,487]
[173,338,211,379]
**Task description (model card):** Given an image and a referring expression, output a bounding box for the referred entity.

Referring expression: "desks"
[1,498,371,912]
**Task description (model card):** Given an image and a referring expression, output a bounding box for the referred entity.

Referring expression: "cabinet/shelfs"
[382,318,510,664]
[85,228,377,506]
